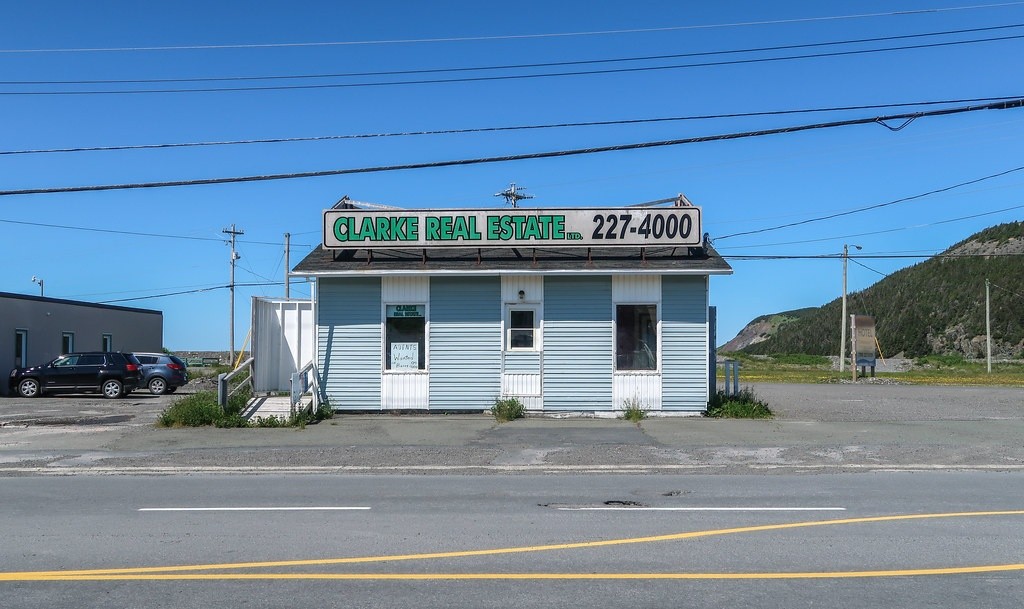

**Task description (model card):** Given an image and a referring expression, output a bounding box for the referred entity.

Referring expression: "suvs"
[132,352,189,395]
[9,352,145,399]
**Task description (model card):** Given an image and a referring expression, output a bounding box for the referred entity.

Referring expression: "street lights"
[840,243,863,373]
[31,276,44,297]
